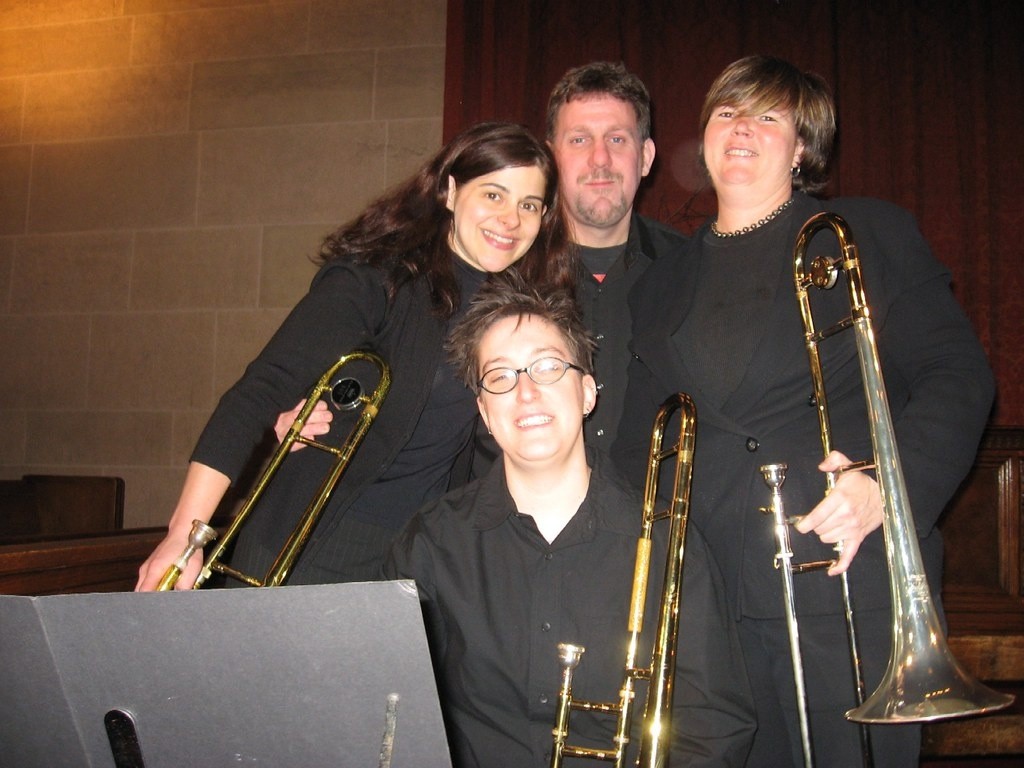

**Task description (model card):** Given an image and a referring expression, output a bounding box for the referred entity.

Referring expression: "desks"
[0,531,167,597]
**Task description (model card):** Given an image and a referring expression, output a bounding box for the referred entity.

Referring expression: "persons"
[133,62,697,594]
[385,271,758,768]
[626,51,992,768]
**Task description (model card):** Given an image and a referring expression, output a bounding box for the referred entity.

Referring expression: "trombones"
[548,387,700,768]
[152,350,396,590]
[757,211,1020,766]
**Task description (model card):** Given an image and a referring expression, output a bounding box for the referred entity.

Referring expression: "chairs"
[0,474,125,534]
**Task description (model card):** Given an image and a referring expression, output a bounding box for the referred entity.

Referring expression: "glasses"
[477,357,587,395]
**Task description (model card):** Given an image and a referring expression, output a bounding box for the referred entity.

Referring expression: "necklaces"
[710,199,797,238]
